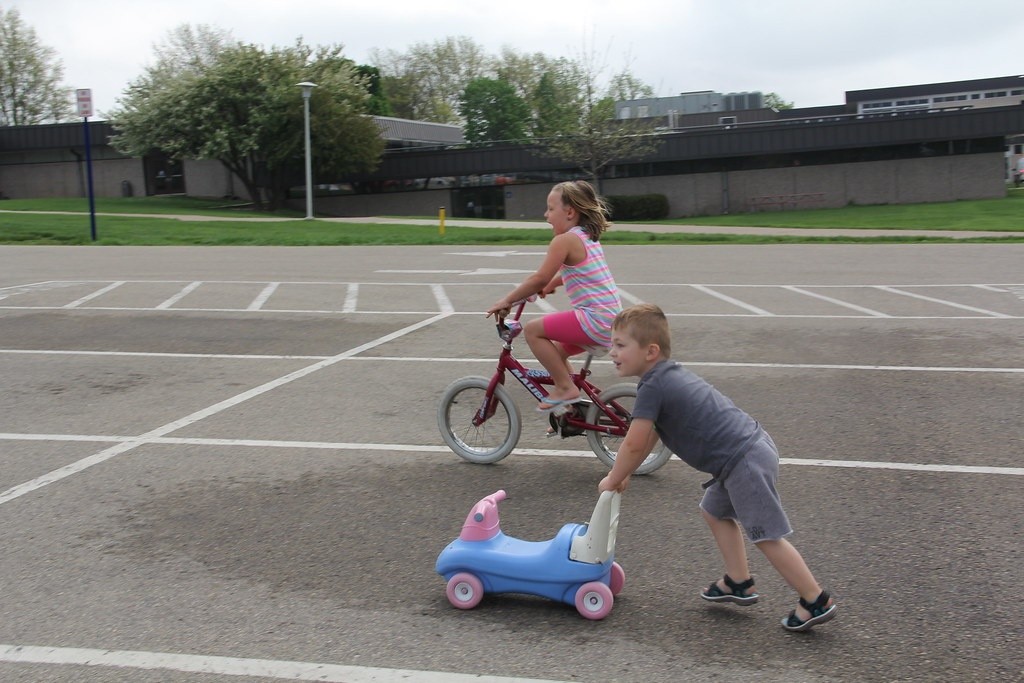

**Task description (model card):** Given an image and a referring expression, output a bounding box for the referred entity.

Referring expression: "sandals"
[701,570,761,606]
[781,588,837,632]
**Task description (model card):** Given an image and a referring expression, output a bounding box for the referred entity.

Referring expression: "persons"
[485,179,626,411]
[597,304,836,630]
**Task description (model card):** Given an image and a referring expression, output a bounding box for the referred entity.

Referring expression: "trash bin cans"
[122,180,131,197]
[449,185,506,219]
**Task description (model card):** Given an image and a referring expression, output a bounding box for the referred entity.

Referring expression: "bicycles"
[437,284,675,476]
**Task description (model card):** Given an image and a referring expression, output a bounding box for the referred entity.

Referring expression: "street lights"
[295,80,318,219]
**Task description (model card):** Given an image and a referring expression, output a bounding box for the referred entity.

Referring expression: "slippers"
[536,395,584,411]
[546,425,560,439]
